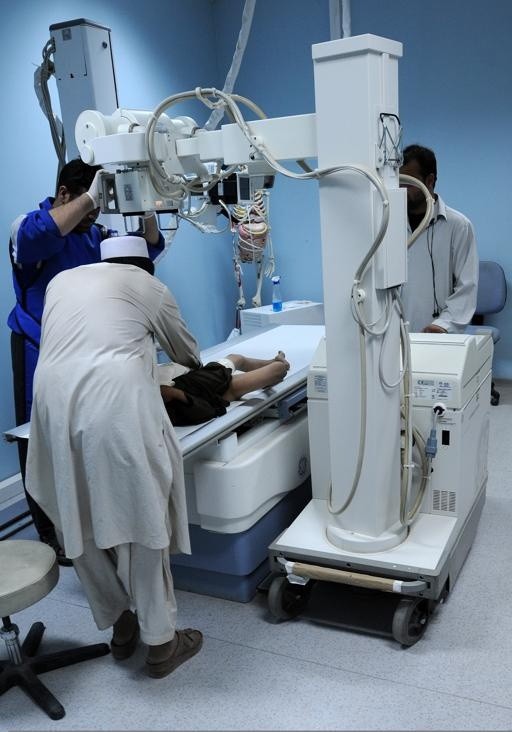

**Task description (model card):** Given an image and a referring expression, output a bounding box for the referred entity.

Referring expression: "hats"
[99,236,150,261]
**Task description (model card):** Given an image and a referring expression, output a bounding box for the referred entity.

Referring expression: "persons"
[5,159,168,565]
[157,348,289,428]
[24,236,203,677]
[399,145,478,332]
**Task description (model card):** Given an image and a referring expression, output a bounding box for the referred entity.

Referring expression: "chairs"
[0,540,110,721]
[466,261,506,406]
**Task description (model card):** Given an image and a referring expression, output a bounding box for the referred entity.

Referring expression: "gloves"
[86,168,113,209]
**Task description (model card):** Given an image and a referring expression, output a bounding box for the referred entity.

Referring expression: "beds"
[5,323,326,461]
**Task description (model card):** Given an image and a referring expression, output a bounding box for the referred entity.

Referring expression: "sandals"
[145,628,203,679]
[39,527,73,567]
[110,615,140,661]
